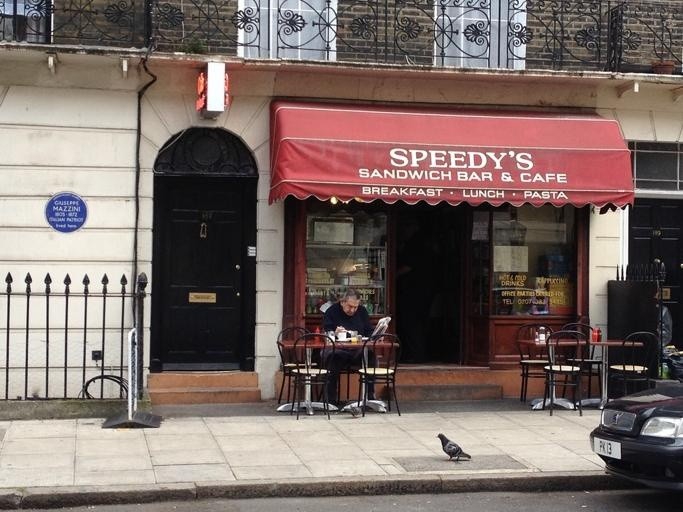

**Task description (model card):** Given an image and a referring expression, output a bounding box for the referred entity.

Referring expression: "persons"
[316,288,388,414]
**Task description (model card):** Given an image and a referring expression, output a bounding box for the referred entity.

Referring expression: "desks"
[518,335,644,410]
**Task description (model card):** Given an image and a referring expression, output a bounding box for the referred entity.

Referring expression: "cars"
[588,382,682,492]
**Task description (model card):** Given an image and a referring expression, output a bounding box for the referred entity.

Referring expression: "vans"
[494,286,544,312]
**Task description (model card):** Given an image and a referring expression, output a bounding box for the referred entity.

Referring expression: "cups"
[591,328,601,342]
[350,331,357,343]
[336,332,345,339]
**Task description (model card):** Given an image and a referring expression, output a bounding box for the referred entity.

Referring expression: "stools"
[324,367,362,407]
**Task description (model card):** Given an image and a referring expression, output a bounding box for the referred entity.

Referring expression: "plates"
[335,338,350,342]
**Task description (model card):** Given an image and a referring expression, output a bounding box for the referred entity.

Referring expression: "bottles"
[534,326,551,342]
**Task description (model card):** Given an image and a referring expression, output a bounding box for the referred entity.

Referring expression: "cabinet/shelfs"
[303,242,386,315]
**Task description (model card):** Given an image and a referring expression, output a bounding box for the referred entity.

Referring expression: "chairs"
[540,329,591,417]
[275,325,316,406]
[560,321,604,403]
[287,333,338,421]
[515,321,557,404]
[356,333,403,418]
[605,330,659,398]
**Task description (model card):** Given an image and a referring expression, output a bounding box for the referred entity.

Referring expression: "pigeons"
[436,433,471,462]
